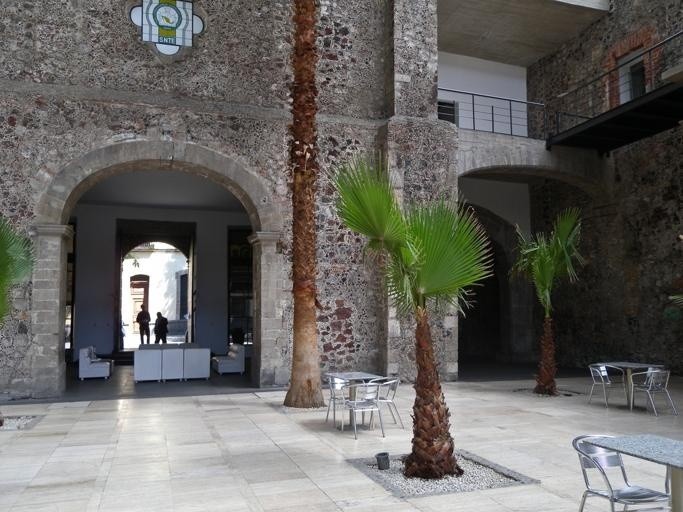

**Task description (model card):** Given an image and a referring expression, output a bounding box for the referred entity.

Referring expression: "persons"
[154,312,168,343]
[136,304,150,344]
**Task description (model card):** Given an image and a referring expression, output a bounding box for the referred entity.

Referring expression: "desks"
[324,371,382,432]
[583,434,682,511]
[604,361,655,409]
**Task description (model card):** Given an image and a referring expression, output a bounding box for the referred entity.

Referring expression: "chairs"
[665,464,670,493]
[326,378,405,439]
[588,362,629,409]
[134,342,211,384]
[630,366,678,417]
[573,434,671,511]
[78,347,111,380]
[87,345,114,375]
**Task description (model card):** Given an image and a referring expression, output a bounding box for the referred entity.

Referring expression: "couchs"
[212,344,245,375]
